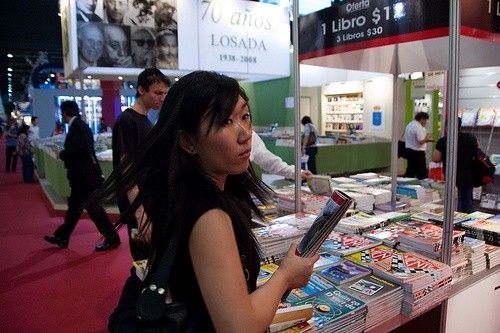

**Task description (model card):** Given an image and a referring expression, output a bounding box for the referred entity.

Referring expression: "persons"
[113,67,171,262]
[42,101,122,251]
[246,127,313,181]
[405,112,431,178]
[0,114,107,183]
[76,0,179,69]
[141,71,320,333]
[431,117,479,214]
[300,115,318,175]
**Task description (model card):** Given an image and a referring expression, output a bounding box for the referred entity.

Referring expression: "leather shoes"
[96,237,121,251]
[44,236,68,248]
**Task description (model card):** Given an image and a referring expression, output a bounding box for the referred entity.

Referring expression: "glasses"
[131,39,155,47]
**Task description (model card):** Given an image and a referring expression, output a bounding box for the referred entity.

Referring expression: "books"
[279,189,353,303]
[249,154,500,333]
[130,228,150,281]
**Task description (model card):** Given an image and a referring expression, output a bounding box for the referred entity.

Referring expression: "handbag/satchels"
[23,147,32,160]
[301,124,316,146]
[108,191,249,333]
[464,149,495,177]
[93,161,105,182]
[301,150,309,171]
[398,140,405,158]
